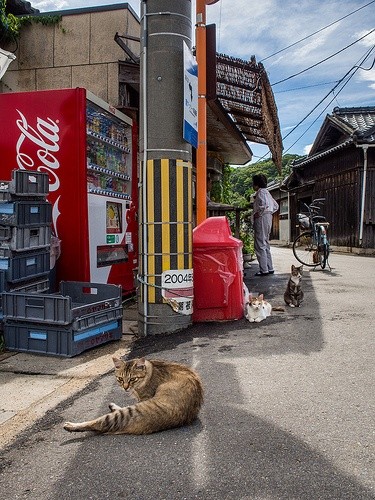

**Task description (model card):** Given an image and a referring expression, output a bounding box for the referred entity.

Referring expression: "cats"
[63,357,204,435]
[284,265,304,307]
[245,293,285,323]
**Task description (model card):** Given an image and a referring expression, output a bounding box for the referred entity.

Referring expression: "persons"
[243,174,279,277]
[207,176,212,202]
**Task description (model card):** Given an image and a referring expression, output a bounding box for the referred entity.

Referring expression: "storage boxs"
[0,170,124,358]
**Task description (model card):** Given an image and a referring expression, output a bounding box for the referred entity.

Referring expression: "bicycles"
[293,198,332,272]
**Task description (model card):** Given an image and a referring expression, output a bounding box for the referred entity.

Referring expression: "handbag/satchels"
[297,213,310,228]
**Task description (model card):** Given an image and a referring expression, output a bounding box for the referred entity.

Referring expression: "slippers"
[268,270,274,274]
[254,271,269,276]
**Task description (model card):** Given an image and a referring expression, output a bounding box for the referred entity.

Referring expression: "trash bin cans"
[192,216,246,323]
